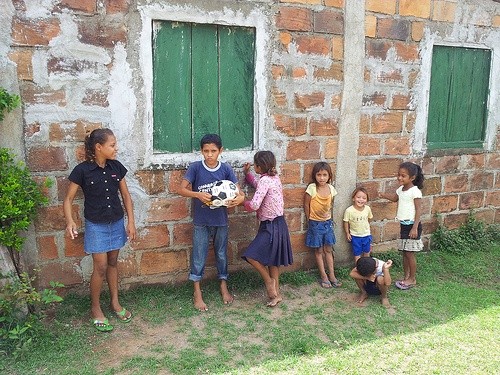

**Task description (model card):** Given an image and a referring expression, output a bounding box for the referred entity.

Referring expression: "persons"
[63,128,137,332]
[344,187,373,264]
[304,162,343,288]
[349,257,393,308]
[178,134,245,312]
[378,161,426,290]
[239,150,294,306]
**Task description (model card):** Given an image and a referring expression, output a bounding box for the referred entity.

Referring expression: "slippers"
[319,278,332,288]
[91,315,113,331]
[112,308,132,323]
[395,280,416,289]
[328,277,342,288]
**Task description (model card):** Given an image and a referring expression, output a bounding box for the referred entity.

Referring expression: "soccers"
[211,180,239,207]
